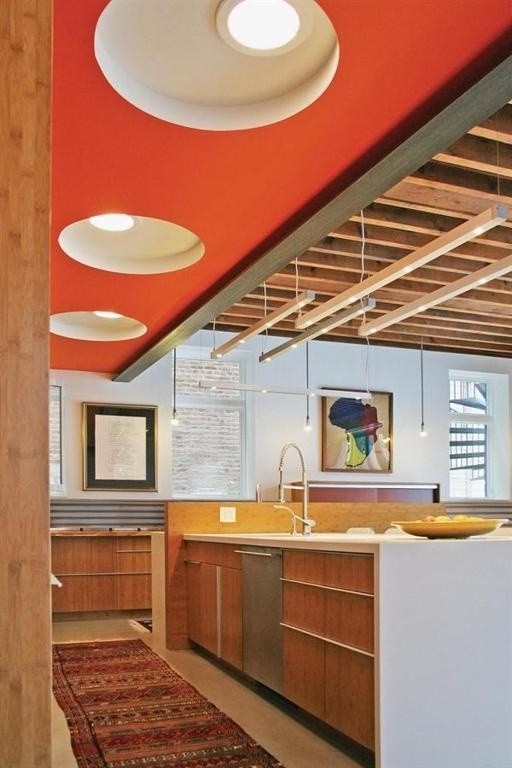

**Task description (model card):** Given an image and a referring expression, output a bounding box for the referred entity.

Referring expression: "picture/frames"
[81,400,159,493]
[318,386,394,475]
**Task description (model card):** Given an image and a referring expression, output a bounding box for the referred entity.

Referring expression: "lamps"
[303,335,314,432]
[254,187,376,364]
[197,317,372,400]
[289,122,507,331]
[207,238,316,358]
[418,334,426,438]
[172,347,182,426]
[352,199,511,339]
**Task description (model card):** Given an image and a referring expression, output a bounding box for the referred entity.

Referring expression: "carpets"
[51,636,287,768]
[130,618,152,636]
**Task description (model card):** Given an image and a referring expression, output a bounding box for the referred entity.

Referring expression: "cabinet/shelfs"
[51,534,115,611]
[114,536,152,611]
[279,545,374,753]
[185,539,244,671]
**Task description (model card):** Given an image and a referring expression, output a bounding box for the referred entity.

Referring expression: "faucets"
[278,442,310,534]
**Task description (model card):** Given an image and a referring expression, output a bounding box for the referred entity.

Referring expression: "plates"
[390,519,510,538]
